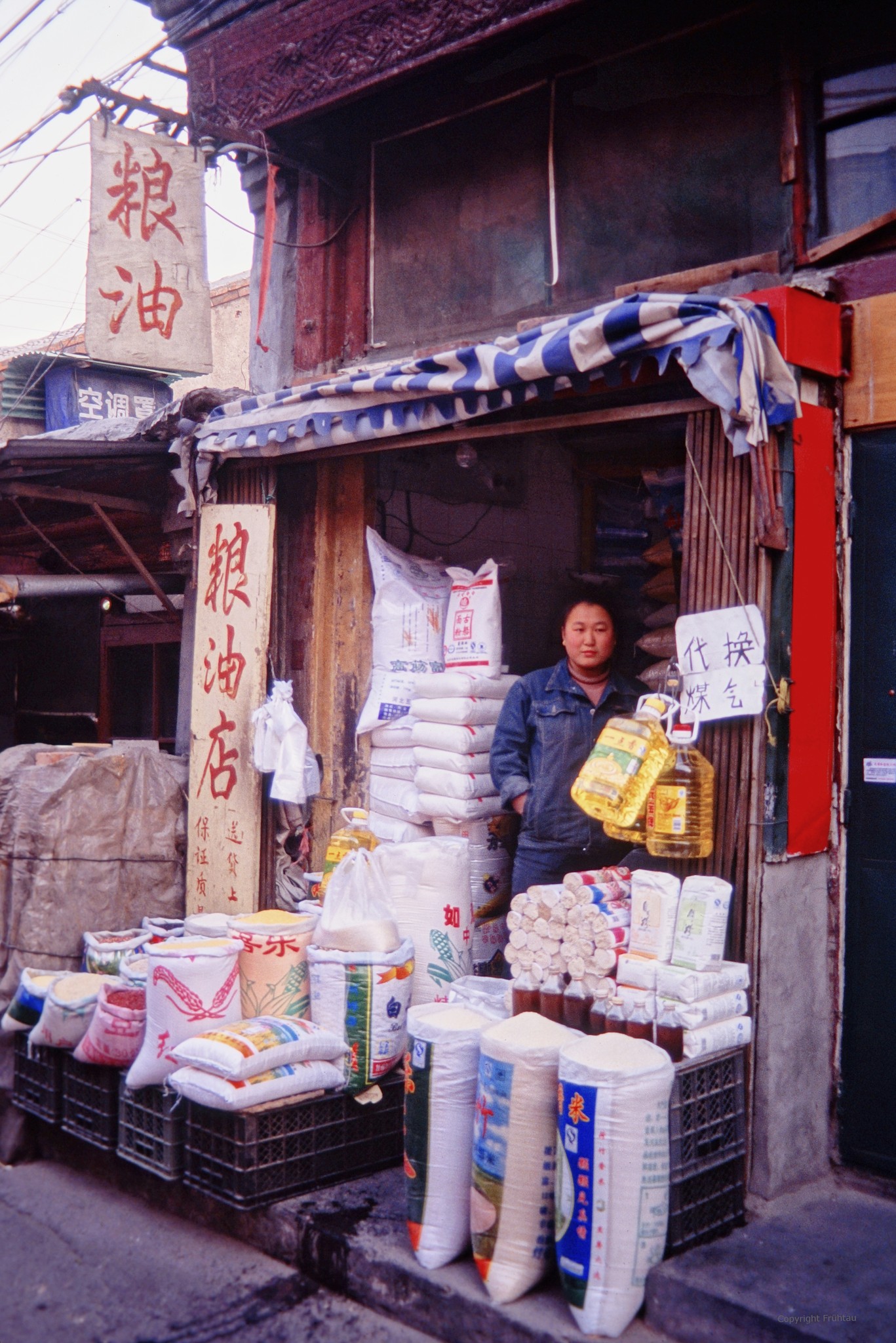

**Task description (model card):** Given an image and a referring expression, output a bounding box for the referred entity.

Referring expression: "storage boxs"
[8,1030,405,1214]
[664,1045,750,1259]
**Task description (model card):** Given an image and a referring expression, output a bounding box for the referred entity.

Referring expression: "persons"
[490,593,657,980]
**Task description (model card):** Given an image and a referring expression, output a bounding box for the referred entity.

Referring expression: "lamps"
[101,596,124,616]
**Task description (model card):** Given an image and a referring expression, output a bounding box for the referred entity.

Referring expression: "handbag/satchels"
[311,848,401,952]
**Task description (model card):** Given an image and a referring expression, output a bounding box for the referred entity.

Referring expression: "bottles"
[317,806,381,905]
[568,691,715,859]
